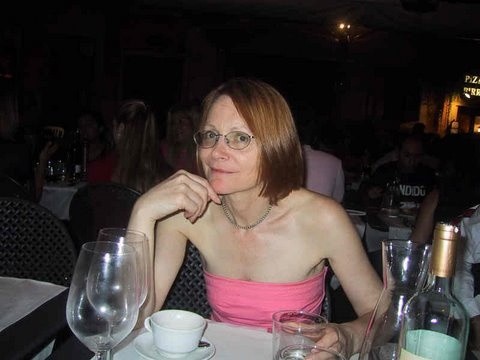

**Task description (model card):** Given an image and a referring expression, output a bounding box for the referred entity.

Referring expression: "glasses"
[193,129,257,151]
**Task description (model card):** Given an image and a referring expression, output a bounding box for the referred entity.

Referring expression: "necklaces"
[220,197,273,228]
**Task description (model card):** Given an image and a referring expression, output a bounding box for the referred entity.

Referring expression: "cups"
[144,310,206,353]
[272,310,328,360]
[37,162,65,184]
[277,344,346,360]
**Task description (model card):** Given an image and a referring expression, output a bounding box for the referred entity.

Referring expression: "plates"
[134,333,216,360]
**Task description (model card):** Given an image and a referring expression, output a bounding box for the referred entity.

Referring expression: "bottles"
[398,223,470,360]
[66,131,90,178]
[358,241,432,360]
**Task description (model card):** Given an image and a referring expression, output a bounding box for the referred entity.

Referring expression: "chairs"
[0,197,79,353]
[70,182,142,254]
[161,238,332,323]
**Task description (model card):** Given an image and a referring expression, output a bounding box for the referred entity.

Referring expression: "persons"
[0,77,58,204]
[125,76,400,360]
[64,107,108,166]
[158,101,201,174]
[293,113,345,202]
[447,204,480,360]
[345,124,377,161]
[407,134,480,243]
[406,123,426,142]
[85,100,172,193]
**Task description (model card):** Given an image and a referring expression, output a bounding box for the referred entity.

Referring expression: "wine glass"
[66,241,139,360]
[87,228,148,360]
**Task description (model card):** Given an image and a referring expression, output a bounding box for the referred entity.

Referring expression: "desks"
[41,181,85,220]
